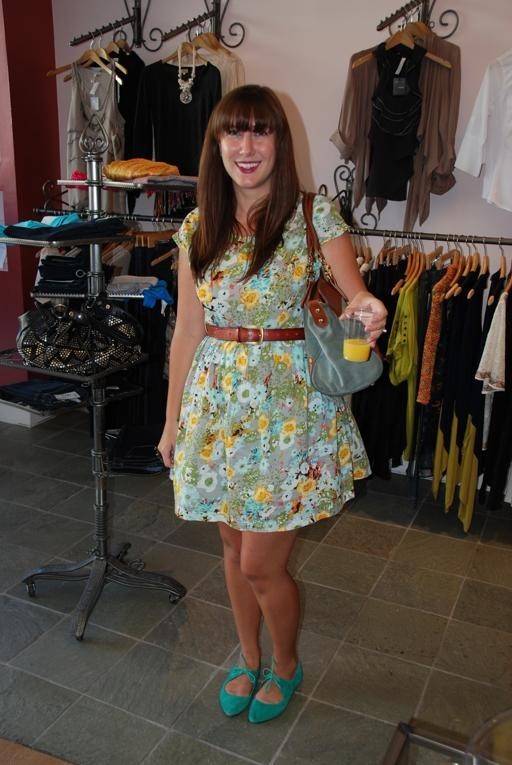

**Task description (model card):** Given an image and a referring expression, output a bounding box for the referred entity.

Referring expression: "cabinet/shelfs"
[0,1,74,392]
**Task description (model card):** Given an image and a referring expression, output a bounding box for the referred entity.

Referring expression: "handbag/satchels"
[298,273,384,396]
[16,294,143,377]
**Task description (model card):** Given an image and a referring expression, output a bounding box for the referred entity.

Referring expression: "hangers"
[100,214,180,267]
[42,15,243,85]
[339,226,512,307]
[348,1,462,70]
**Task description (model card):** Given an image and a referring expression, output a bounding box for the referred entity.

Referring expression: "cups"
[343,303,374,363]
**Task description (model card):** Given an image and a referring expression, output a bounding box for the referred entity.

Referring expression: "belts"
[207,324,307,341]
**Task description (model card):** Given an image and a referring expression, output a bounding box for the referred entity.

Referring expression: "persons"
[157,82,391,724]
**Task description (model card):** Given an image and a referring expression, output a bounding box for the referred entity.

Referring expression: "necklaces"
[174,39,196,105]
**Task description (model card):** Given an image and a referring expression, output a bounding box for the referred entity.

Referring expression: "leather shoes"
[220,652,259,717]
[249,660,302,723]
